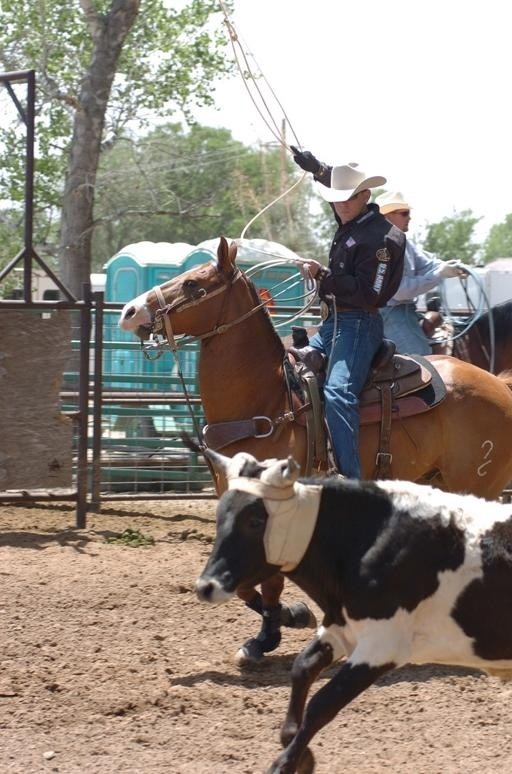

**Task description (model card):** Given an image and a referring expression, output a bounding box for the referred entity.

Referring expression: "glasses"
[394,211,410,216]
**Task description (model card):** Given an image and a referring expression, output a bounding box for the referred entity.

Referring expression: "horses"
[119,236,512,670]
[278,298,512,388]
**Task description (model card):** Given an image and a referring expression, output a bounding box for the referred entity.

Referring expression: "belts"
[329,301,379,312]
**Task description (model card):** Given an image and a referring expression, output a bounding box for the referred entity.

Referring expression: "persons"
[425,284,453,309]
[289,144,407,480]
[369,191,471,357]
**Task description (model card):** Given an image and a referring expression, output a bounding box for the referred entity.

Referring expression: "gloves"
[290,145,321,174]
[433,259,468,279]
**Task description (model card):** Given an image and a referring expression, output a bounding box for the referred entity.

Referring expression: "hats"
[317,161,388,202]
[375,190,414,215]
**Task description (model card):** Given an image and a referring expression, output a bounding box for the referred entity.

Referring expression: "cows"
[194,445,512,774]
